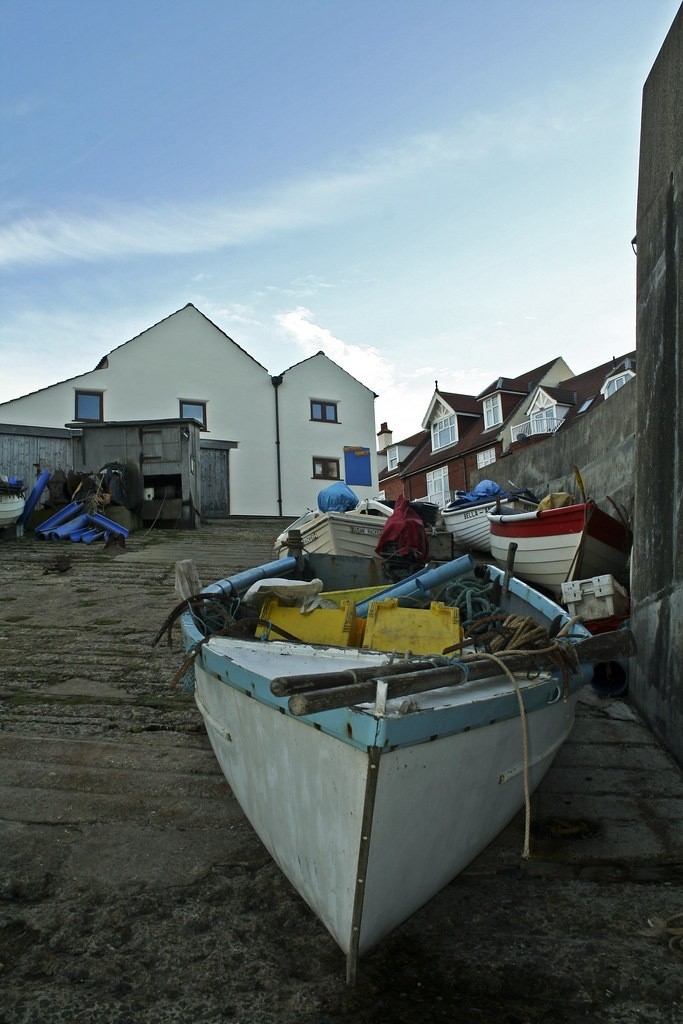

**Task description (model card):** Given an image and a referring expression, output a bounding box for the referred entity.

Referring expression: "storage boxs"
[561,575,630,634]
[255,586,464,657]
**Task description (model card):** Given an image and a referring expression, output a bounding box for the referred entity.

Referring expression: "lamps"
[183,428,190,438]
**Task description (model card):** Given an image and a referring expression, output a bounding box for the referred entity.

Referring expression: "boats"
[274,499,454,564]
[441,490,539,552]
[178,552,596,983]
[487,496,633,603]
